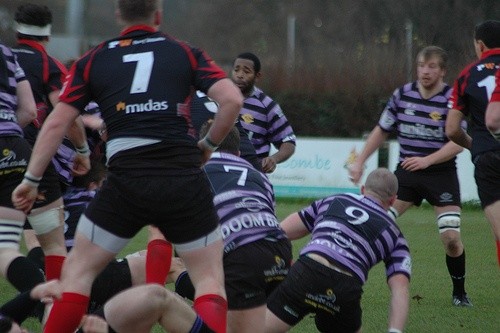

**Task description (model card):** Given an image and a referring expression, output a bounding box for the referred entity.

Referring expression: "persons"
[444,20,500,266]
[351,46,472,306]
[0,0,295,333]
[484,68,500,142]
[264,167,412,333]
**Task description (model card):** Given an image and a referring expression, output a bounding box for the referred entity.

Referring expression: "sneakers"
[452,293,474,306]
